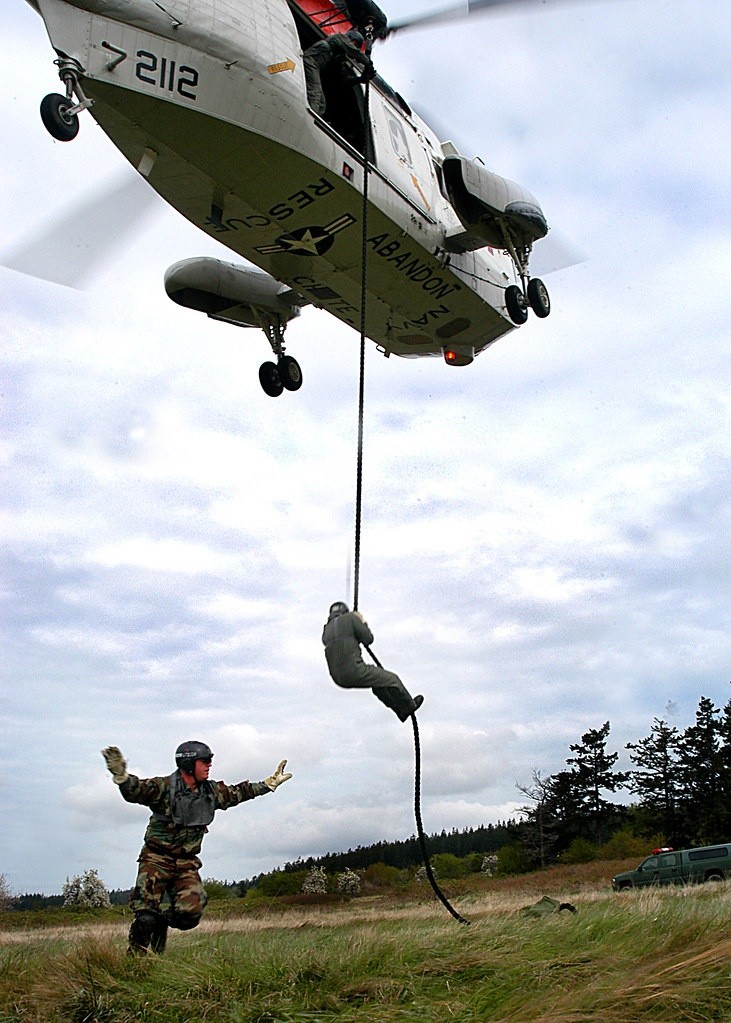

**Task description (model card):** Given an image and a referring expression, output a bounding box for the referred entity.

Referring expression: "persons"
[322,601,424,722]
[304,31,377,124]
[101,741,293,957]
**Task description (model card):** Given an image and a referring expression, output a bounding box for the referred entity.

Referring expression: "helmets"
[175,741,214,774]
[328,602,349,619]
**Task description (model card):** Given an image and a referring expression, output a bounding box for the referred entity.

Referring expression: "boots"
[151,911,203,955]
[126,911,155,958]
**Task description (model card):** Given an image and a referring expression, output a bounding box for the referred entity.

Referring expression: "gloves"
[265,760,292,792]
[102,746,129,785]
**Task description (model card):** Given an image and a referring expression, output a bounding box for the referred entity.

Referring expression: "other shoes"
[397,695,424,723]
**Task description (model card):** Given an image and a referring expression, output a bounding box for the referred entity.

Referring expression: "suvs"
[612,842,731,893]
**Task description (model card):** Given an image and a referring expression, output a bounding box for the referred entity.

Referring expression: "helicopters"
[26,0,552,396]
